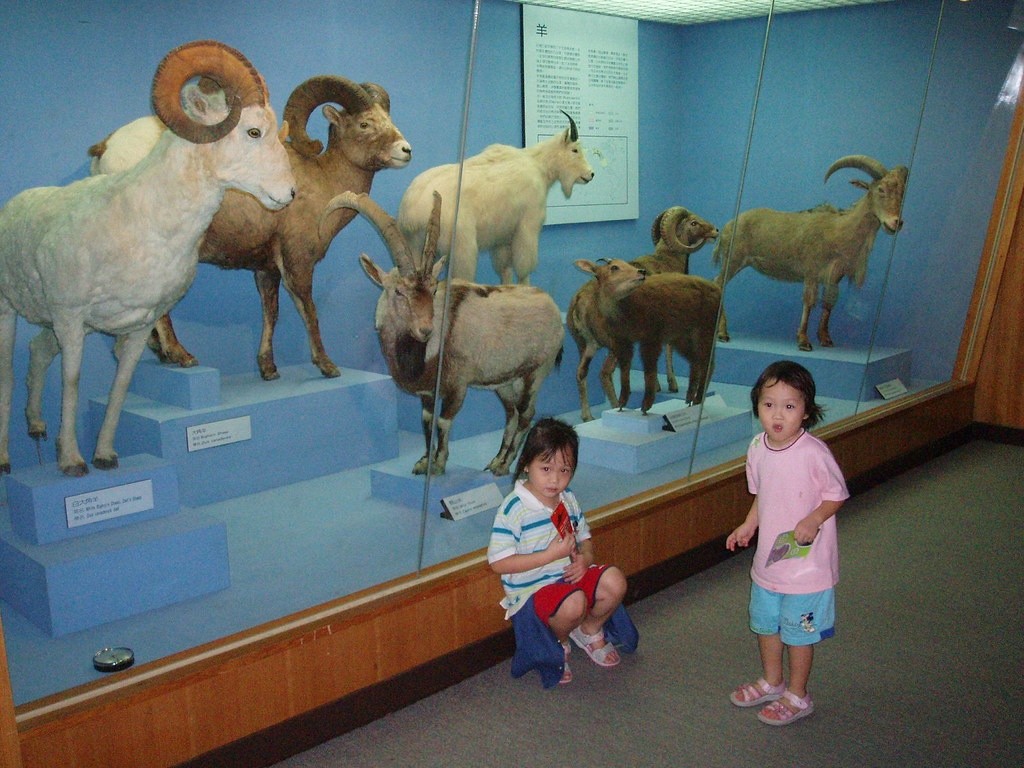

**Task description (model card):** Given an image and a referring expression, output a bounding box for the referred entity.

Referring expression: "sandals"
[559,641,573,684]
[569,623,621,666]
[731,676,787,707]
[757,690,815,726]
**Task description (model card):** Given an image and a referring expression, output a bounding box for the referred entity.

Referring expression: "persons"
[726,360,850,725]
[486,419,627,683]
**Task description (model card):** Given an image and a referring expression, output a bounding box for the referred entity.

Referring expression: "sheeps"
[317,191,566,477]
[88,76,414,381]
[396,110,595,289]
[565,206,723,422]
[710,154,909,353]
[0,39,296,477]
